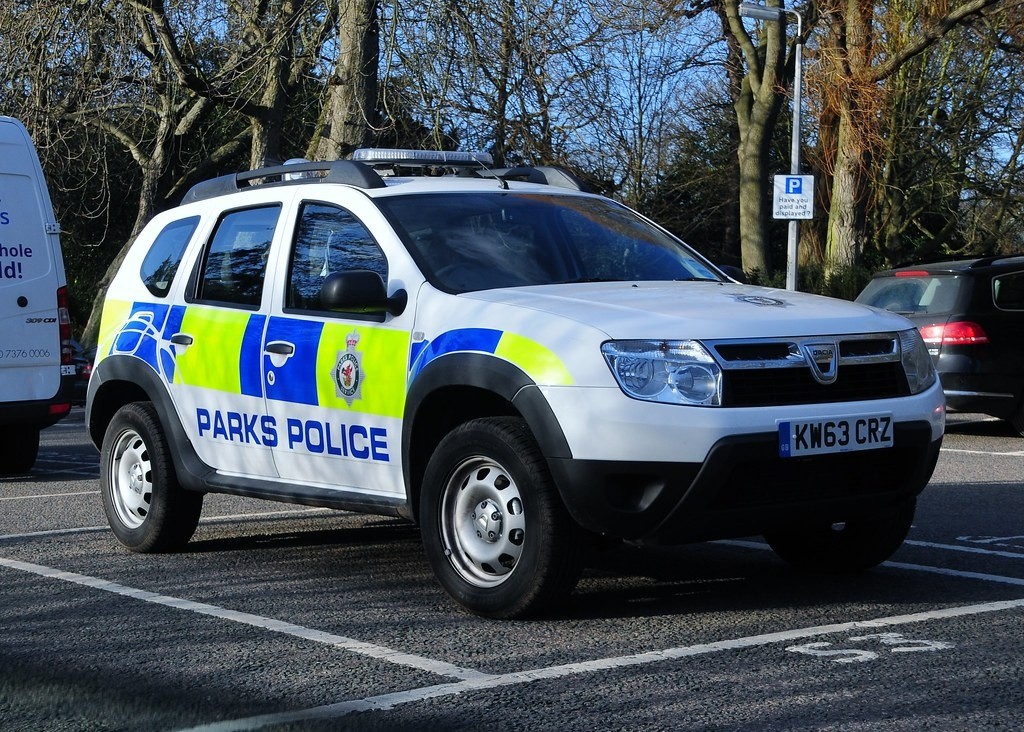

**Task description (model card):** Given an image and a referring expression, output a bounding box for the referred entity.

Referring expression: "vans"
[0,113,72,472]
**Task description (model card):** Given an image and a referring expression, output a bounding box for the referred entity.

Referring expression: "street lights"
[738,2,814,292]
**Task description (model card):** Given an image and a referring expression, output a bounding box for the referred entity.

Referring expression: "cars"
[71,338,97,407]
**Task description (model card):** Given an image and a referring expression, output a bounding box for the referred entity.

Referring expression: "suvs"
[853,252,1023,438]
[84,148,947,619]
